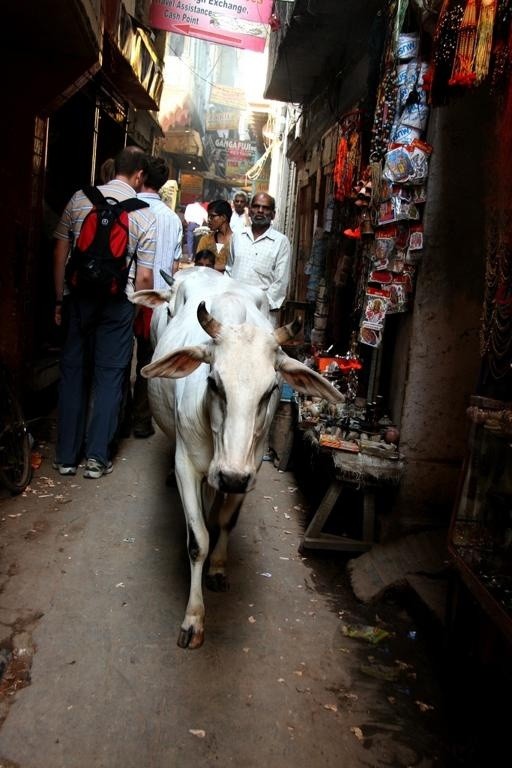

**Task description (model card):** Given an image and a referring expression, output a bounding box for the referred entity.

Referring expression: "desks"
[278,393,406,557]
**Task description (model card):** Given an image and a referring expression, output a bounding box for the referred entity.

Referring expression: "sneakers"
[52,461,77,475]
[83,458,114,479]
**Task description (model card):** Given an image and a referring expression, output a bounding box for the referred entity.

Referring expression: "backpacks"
[62,186,150,299]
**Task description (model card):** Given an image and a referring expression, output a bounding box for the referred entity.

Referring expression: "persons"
[194,199,236,276]
[49,145,160,480]
[229,190,254,234]
[213,190,293,321]
[193,249,217,270]
[100,152,187,439]
[183,196,209,266]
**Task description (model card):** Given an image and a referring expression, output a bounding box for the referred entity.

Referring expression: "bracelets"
[54,297,64,306]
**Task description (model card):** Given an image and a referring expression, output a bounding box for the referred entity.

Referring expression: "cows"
[128,266,228,350]
[140,271,346,649]
